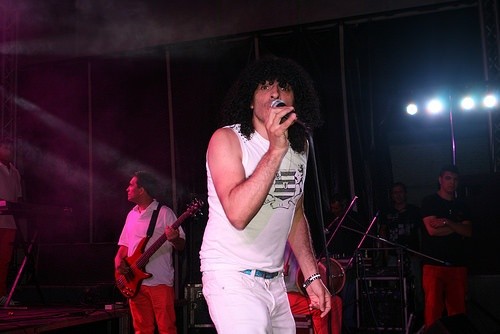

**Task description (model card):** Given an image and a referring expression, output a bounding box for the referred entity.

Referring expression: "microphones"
[271,99,312,134]
[324,216,340,234]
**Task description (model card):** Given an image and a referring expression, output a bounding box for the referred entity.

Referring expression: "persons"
[0,138,23,313]
[195,56,334,334]
[415,165,473,334]
[375,180,429,309]
[319,191,367,257]
[113,171,187,334]
[281,233,345,334]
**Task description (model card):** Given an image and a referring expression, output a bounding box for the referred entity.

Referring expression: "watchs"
[442,218,448,225]
[302,272,323,288]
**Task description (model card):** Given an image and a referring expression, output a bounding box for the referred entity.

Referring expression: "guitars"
[111,197,205,300]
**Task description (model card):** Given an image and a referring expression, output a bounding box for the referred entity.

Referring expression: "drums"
[294,258,347,297]
